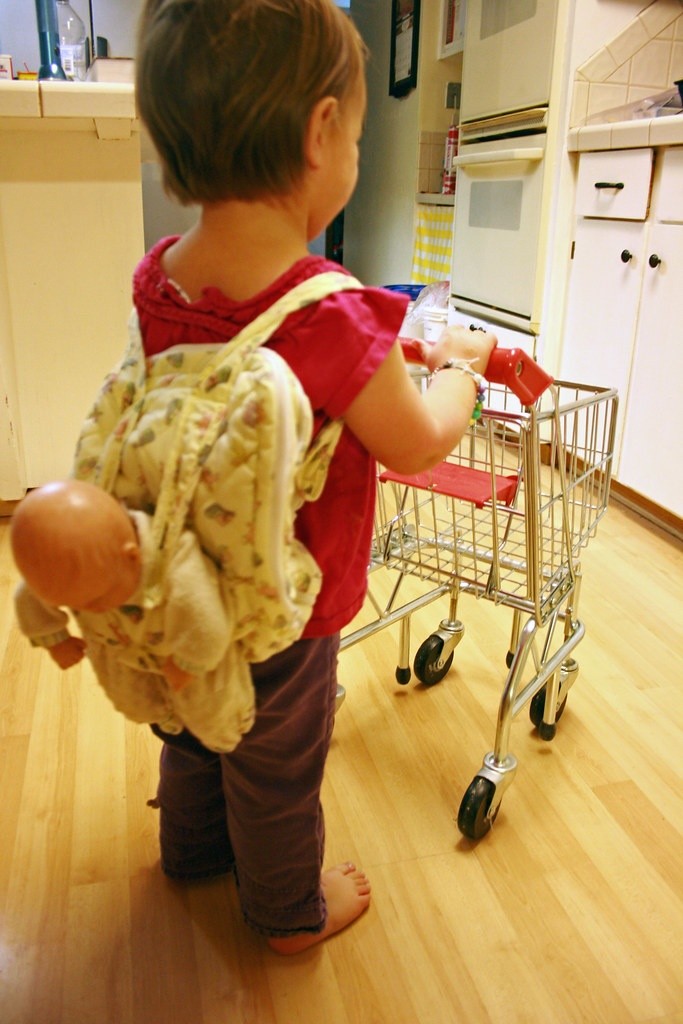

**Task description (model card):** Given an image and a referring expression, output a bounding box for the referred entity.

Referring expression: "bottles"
[55,0,87,81]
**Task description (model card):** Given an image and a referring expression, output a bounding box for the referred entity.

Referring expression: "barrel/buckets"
[423,305,448,347]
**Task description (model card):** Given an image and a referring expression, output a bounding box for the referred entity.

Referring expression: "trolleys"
[335,335,618,841]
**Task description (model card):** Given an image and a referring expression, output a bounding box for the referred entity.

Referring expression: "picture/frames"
[388,0,421,99]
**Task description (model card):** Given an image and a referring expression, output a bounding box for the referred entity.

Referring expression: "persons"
[131,0,497,954]
[9,480,255,755]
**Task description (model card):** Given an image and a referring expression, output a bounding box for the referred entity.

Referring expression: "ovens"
[448,132,551,435]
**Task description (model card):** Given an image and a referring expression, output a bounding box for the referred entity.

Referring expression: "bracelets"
[431,358,484,425]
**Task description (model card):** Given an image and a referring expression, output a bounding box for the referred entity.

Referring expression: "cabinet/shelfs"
[555,144,683,541]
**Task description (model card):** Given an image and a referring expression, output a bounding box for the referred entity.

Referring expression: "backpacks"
[76,271,362,661]
